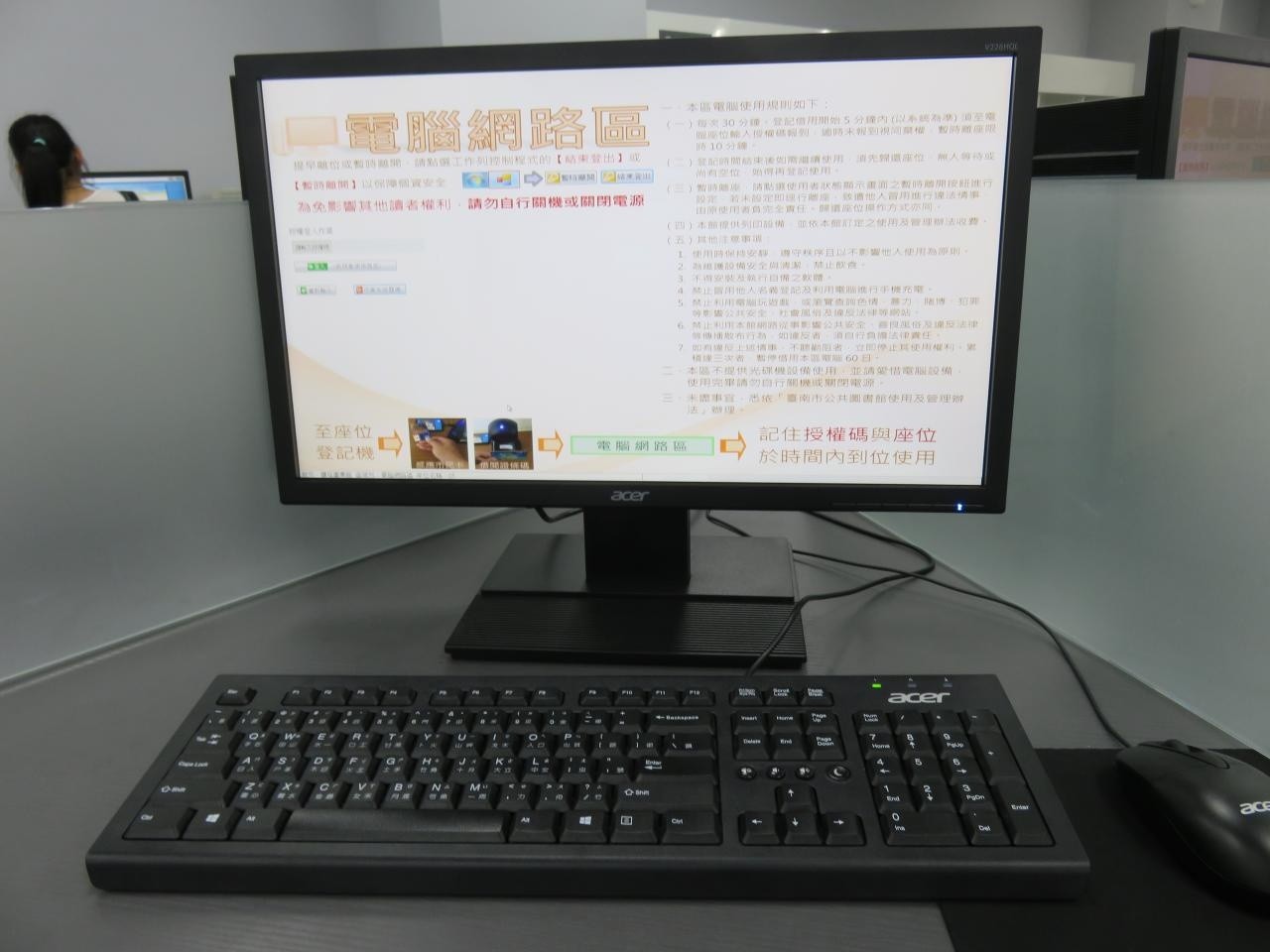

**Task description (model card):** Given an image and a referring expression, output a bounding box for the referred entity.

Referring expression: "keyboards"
[85,672,1084,900]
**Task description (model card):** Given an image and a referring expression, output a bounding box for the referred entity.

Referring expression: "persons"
[9,111,147,210]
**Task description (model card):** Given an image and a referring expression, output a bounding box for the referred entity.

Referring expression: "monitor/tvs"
[1132,26,1269,184]
[232,25,1046,668]
[82,169,192,205]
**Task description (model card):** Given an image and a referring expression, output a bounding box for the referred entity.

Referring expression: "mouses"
[1116,735,1270,913]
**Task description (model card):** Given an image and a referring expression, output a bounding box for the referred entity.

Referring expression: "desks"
[0,506,1270,952]
